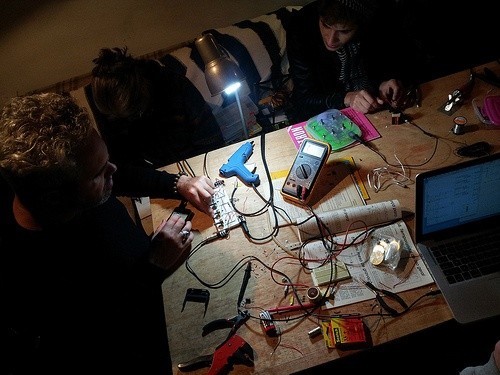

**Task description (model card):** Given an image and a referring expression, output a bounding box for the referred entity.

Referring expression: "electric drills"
[219,140,260,187]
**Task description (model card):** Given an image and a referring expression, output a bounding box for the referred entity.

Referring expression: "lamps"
[195,32,249,140]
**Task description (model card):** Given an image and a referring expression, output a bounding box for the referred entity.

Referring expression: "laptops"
[414,153,500,324]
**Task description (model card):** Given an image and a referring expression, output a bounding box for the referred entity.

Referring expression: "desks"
[150,59,500,375]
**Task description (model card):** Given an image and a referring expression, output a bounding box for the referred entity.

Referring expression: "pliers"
[358,276,408,315]
[202,310,250,349]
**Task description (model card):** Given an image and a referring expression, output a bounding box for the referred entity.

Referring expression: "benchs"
[19,5,306,147]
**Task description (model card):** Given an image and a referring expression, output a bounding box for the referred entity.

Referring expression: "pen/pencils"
[237,262,251,307]
[265,303,315,312]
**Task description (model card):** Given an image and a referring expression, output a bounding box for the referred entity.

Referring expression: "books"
[263,155,370,229]
[286,107,381,155]
[296,199,434,311]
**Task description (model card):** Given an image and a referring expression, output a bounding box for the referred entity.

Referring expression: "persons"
[286,0,427,117]
[84,46,226,167]
[0,91,215,375]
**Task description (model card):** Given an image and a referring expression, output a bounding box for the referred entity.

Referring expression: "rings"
[179,229,190,241]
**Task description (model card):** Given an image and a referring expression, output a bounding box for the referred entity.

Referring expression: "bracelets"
[173,171,188,196]
